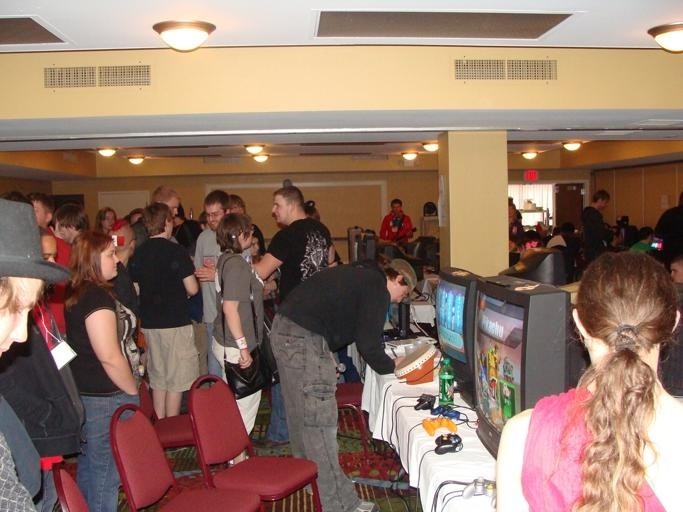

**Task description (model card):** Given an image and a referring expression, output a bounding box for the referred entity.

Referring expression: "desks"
[346,302,498,511]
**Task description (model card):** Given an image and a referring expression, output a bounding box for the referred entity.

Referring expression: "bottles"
[500,370,515,424]
[439,359,454,404]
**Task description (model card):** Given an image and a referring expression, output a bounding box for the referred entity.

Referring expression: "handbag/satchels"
[224,346,278,399]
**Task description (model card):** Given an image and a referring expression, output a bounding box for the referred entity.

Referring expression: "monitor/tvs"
[474,274,592,461]
[435,267,485,411]
[358,233,385,264]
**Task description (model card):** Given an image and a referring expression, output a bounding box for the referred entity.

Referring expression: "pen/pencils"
[391,348,397,358]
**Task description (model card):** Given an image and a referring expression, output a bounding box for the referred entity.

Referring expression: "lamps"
[421,143,438,152]
[152,21,216,53]
[95,147,119,157]
[646,22,682,52]
[400,152,418,160]
[561,142,581,151]
[127,156,145,165]
[243,144,265,154]
[252,155,269,162]
[520,151,538,160]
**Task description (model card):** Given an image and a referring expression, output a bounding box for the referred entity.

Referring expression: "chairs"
[186,374,323,512]
[53,463,90,512]
[137,377,224,471]
[110,402,265,512]
[335,381,369,460]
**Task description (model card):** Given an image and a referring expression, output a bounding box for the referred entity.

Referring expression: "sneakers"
[353,501,380,512]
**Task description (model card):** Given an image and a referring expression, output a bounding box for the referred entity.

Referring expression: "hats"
[0,197,70,283]
[389,258,417,304]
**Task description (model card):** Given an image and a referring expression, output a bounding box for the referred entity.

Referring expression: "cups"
[404,345,418,356]
[413,341,425,351]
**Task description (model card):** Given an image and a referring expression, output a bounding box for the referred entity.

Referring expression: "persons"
[270,258,417,512]
[0,187,362,510]
[508,187,683,284]
[379,198,413,247]
[495,251,683,512]
